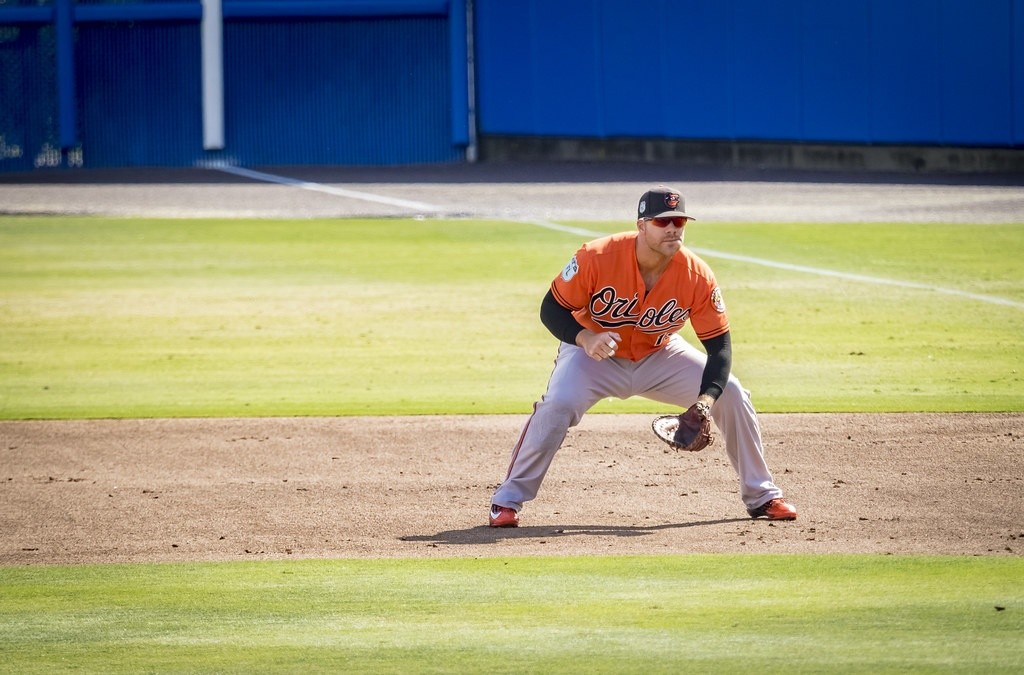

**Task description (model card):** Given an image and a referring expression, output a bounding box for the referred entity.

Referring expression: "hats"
[638,185,696,221]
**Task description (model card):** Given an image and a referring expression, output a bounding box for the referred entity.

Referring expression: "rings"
[608,340,615,348]
[608,350,614,356]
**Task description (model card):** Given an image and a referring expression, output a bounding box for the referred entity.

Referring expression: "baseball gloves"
[651,401,716,453]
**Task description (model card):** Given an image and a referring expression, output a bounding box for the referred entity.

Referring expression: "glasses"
[639,217,688,228]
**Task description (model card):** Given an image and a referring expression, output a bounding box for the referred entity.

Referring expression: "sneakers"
[489,504,519,527]
[747,498,797,520]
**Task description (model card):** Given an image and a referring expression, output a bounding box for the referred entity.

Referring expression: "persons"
[489,184,796,528]
